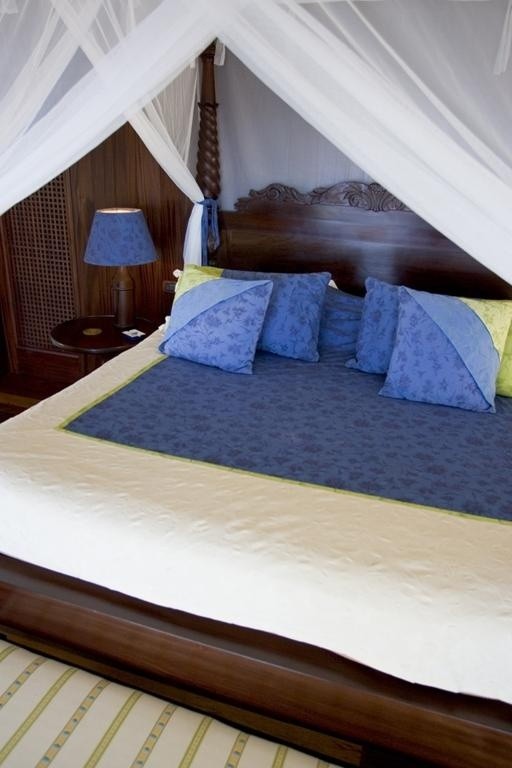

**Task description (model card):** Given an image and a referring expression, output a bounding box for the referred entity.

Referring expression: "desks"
[50,317,158,378]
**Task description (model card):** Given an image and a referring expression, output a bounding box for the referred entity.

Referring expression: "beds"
[1,41,512,768]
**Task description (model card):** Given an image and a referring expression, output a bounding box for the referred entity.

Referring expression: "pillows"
[379,286,512,413]
[195,266,332,362]
[159,267,274,375]
[344,277,512,399]
[318,286,365,355]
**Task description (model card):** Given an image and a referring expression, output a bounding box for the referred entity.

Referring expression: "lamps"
[82,208,159,329]
